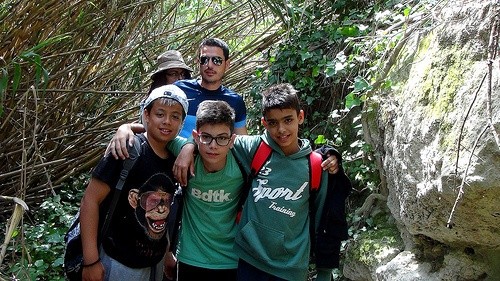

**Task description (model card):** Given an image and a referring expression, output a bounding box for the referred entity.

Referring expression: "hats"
[144,84,189,120]
[149,50,193,81]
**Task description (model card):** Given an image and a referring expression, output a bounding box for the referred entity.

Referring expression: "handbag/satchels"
[63,208,86,281]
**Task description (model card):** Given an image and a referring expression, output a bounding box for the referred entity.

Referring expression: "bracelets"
[83,257,101,267]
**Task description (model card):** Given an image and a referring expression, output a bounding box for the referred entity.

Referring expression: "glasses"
[198,56,223,67]
[197,133,231,147]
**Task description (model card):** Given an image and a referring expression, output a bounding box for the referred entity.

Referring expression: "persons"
[104,99,338,281]
[80,85,189,281]
[139,50,193,121]
[170,38,248,139]
[172,83,340,281]
